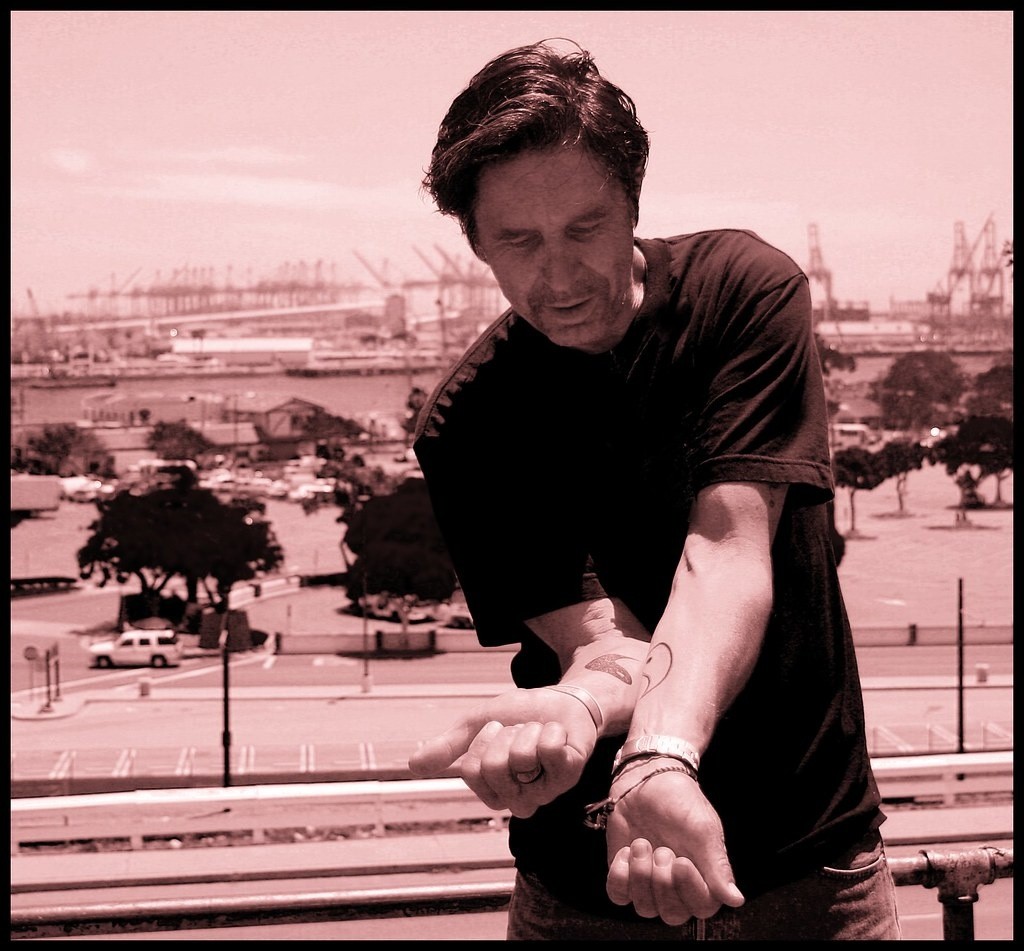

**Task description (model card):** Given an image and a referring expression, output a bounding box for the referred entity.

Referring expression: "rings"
[515,765,543,785]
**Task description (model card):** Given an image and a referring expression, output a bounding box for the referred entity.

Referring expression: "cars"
[63,441,430,506]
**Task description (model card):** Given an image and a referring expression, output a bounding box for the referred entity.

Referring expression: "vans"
[830,422,873,446]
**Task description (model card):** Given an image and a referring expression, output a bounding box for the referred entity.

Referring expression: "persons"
[407,35,904,946]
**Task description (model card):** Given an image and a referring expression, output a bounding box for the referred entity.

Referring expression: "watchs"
[611,733,703,774]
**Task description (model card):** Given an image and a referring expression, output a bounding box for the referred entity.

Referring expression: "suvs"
[86,627,187,667]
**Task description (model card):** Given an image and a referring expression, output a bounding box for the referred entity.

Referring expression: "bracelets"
[544,684,606,741]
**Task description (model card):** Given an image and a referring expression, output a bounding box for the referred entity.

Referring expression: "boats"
[29,339,128,392]
